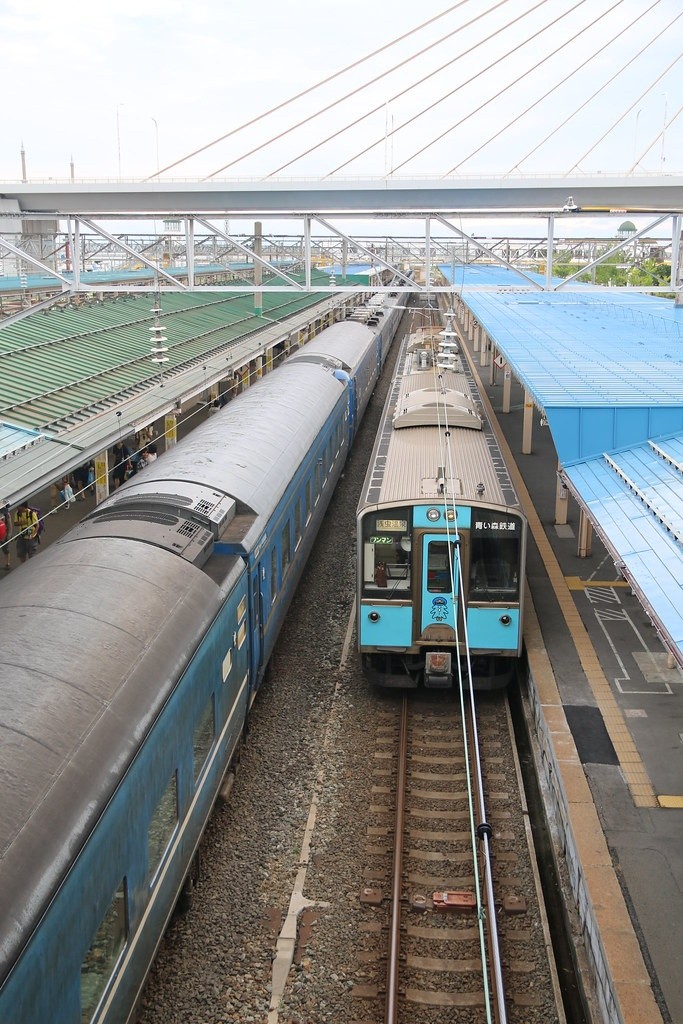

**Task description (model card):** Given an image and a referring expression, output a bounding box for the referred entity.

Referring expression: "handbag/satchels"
[123,464,134,480]
[88,468,95,485]
[59,484,76,502]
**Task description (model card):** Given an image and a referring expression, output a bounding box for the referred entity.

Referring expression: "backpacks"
[17,506,44,536]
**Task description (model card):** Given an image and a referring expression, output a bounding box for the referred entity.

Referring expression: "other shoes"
[5,563,11,571]
[48,487,94,514]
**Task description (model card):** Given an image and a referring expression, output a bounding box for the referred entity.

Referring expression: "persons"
[12,498,39,565]
[48,421,158,521]
[0,507,13,571]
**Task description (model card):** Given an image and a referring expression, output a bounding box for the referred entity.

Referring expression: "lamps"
[425,506,441,522]
[445,508,458,522]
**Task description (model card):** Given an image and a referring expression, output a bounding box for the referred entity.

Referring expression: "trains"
[356,329,529,690]
[0,271,416,1023]
